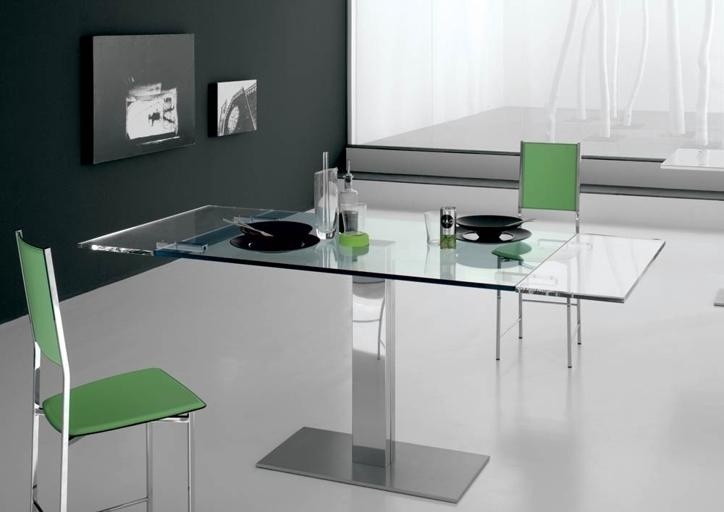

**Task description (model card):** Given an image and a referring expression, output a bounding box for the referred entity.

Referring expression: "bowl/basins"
[237,220,313,246]
[455,215,522,238]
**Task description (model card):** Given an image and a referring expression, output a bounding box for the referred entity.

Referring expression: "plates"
[453,225,531,245]
[229,236,319,253]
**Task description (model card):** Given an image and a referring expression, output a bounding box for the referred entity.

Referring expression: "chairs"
[14,228,205,509]
[492,140,582,369]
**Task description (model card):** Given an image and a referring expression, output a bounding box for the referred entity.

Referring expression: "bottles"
[338,173,357,232]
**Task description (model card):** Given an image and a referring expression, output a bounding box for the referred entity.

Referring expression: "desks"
[656,146,724,308]
[75,206,668,504]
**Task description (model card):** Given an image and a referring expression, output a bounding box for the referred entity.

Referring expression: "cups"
[313,168,338,239]
[355,202,367,232]
[423,210,438,244]
